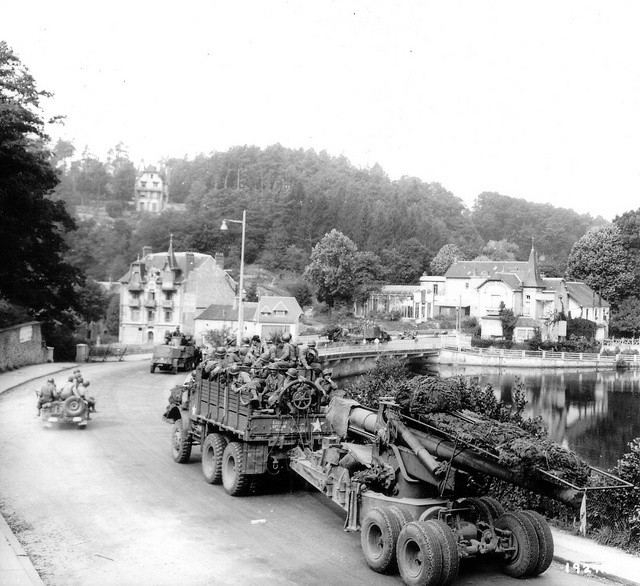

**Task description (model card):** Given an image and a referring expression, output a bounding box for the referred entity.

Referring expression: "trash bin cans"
[46,345,54,362]
[75,343,87,361]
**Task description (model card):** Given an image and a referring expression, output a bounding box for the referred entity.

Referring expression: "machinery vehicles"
[150,335,196,375]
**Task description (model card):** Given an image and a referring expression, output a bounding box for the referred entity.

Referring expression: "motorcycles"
[35,396,88,430]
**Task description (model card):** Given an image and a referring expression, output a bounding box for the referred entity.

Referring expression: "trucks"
[163,365,341,498]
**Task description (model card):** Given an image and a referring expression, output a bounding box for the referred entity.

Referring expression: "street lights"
[220,209,247,348]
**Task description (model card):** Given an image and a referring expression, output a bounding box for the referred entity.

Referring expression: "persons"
[209,333,338,404]
[37,378,59,418]
[59,370,96,413]
[165,328,196,346]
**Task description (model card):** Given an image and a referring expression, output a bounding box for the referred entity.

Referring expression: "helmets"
[48,378,54,383]
[307,339,316,346]
[322,369,331,378]
[241,336,249,343]
[83,381,89,387]
[280,333,291,343]
[240,349,247,355]
[228,365,240,375]
[227,335,236,345]
[68,376,73,382]
[77,378,84,383]
[259,353,269,362]
[268,364,278,370]
[74,369,80,373]
[216,346,225,356]
[75,374,81,379]
[285,368,297,378]
